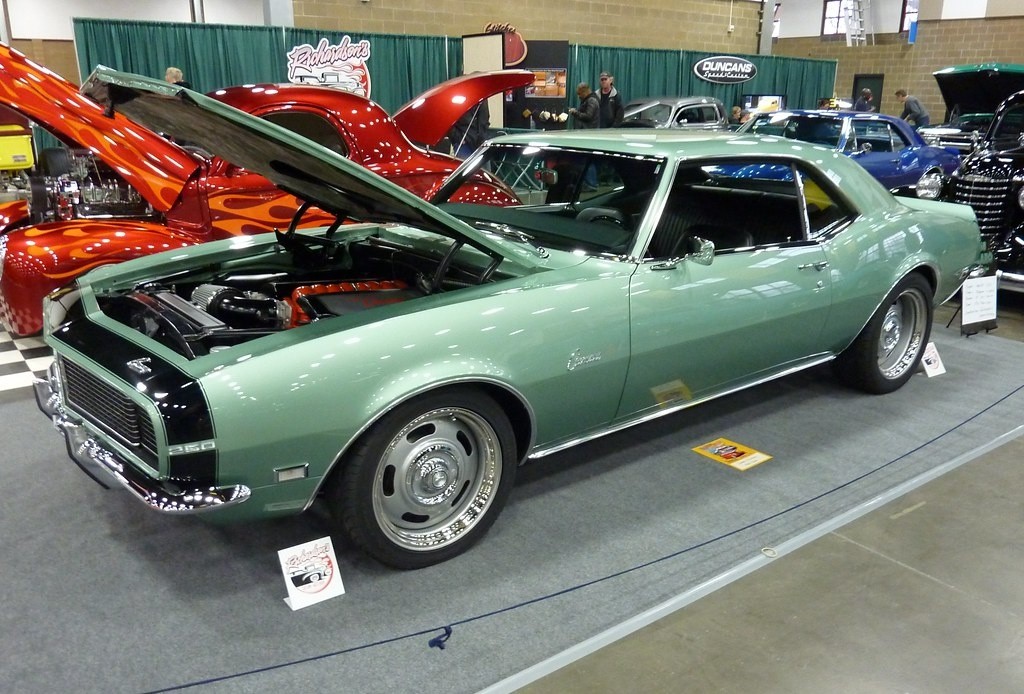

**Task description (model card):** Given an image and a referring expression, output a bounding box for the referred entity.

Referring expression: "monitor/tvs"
[741,94,787,118]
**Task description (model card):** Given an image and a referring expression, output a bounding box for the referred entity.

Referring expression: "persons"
[569,83,600,193]
[595,71,624,128]
[818,100,831,110]
[728,107,750,125]
[895,89,929,129]
[162,67,191,146]
[449,71,490,159]
[855,89,873,112]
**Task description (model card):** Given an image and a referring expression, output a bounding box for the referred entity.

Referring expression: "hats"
[600,72,614,85]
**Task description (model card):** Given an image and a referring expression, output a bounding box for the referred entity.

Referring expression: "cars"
[915,61,1024,158]
[625,93,730,133]
[29,63,986,571]
[0,40,538,340]
[733,109,961,201]
[934,89,1024,298]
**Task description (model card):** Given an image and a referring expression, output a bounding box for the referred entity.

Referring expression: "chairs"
[683,112,698,123]
[575,206,635,231]
[669,222,755,256]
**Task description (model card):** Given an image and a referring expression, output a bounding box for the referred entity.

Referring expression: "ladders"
[843,2,867,46]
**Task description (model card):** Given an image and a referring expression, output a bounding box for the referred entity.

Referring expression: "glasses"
[601,78,607,81]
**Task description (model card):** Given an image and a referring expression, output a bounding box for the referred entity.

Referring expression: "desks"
[489,126,544,191]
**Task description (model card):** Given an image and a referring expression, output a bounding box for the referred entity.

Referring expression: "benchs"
[673,182,802,243]
